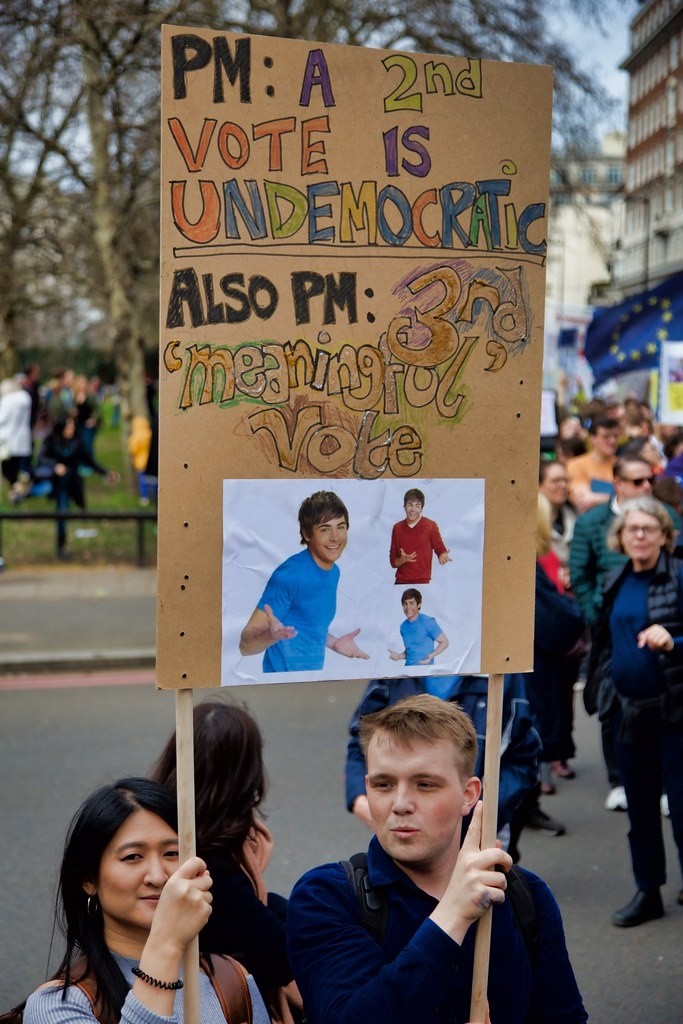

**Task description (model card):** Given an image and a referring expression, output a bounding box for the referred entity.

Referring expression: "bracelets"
[131,967,184,990]
[332,639,338,650]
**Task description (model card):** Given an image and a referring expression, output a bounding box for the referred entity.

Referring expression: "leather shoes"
[612,888,666,926]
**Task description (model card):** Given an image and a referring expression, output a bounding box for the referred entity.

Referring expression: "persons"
[387,588,449,667]
[400,654,403,658]
[1,364,683,1024]
[238,490,369,674]
[389,488,453,584]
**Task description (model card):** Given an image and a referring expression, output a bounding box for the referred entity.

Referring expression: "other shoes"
[549,760,576,780]
[540,783,555,795]
[660,794,670,817]
[604,786,627,811]
[523,809,566,837]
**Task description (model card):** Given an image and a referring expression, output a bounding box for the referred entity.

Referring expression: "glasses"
[622,523,662,535]
[616,474,656,487]
